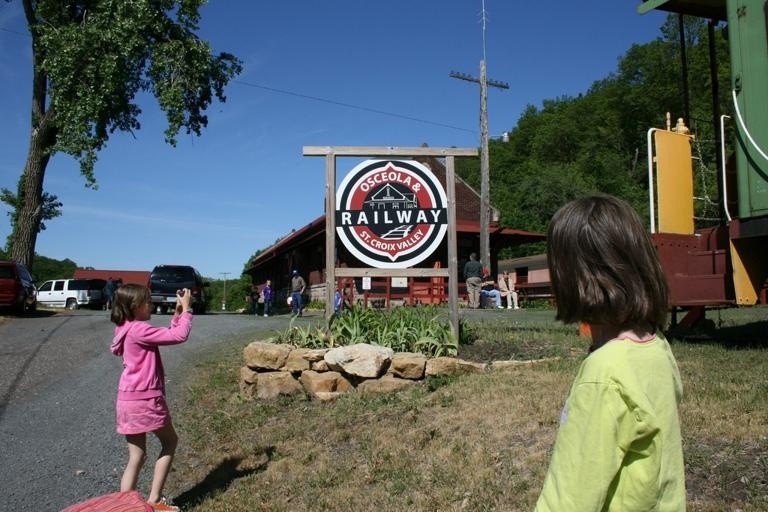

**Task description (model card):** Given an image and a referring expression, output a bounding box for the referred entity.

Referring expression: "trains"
[498,252,556,300]
[636,0,768,341]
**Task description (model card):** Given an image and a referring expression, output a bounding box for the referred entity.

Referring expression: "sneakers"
[291,313,302,317]
[497,305,519,310]
[263,313,268,318]
[144,496,181,512]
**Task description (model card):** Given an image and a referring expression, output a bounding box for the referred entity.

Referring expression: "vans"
[0,260,35,316]
[144,264,207,313]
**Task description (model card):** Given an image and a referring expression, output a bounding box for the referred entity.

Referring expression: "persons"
[530,194,685,511]
[251,285,260,317]
[290,269,306,317]
[255,289,264,315]
[103,276,114,311]
[499,268,519,310]
[262,280,273,316]
[106,284,194,512]
[463,252,482,308]
[480,265,505,311]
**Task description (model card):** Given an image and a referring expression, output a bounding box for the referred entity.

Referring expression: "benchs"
[443,280,556,300]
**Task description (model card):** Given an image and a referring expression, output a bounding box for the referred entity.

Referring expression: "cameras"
[178,291,185,297]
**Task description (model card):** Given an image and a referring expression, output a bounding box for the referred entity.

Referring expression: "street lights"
[478,130,511,277]
[218,272,233,310]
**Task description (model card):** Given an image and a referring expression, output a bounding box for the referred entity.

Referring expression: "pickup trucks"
[34,278,105,309]
